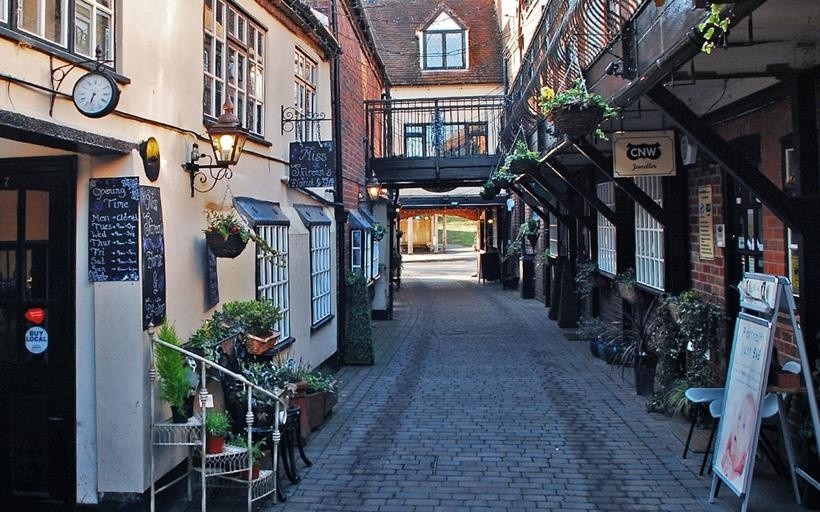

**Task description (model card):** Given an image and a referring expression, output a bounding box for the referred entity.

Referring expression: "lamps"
[365,168,383,202]
[180,92,250,198]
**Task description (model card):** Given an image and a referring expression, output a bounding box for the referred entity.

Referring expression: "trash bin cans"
[482,252,500,284]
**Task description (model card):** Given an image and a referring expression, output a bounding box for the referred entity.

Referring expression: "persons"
[720,392,758,481]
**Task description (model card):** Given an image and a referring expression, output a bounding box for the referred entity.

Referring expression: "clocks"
[47,43,121,118]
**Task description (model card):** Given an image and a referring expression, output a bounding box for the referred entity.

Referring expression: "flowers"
[538,82,617,115]
[203,209,280,242]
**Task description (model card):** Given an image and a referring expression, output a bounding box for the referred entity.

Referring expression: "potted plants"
[480,122,541,201]
[576,262,716,422]
[154,297,340,502]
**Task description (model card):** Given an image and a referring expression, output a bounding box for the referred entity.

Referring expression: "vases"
[552,107,604,138]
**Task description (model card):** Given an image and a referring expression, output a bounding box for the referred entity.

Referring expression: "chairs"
[683,387,725,474]
[700,362,803,498]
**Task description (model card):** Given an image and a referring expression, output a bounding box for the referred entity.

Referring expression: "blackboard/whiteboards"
[89,177,166,330]
[206,237,220,307]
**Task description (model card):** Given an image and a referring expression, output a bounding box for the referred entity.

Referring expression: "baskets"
[557,108,604,136]
[181,331,239,371]
[304,380,339,429]
[483,159,532,200]
